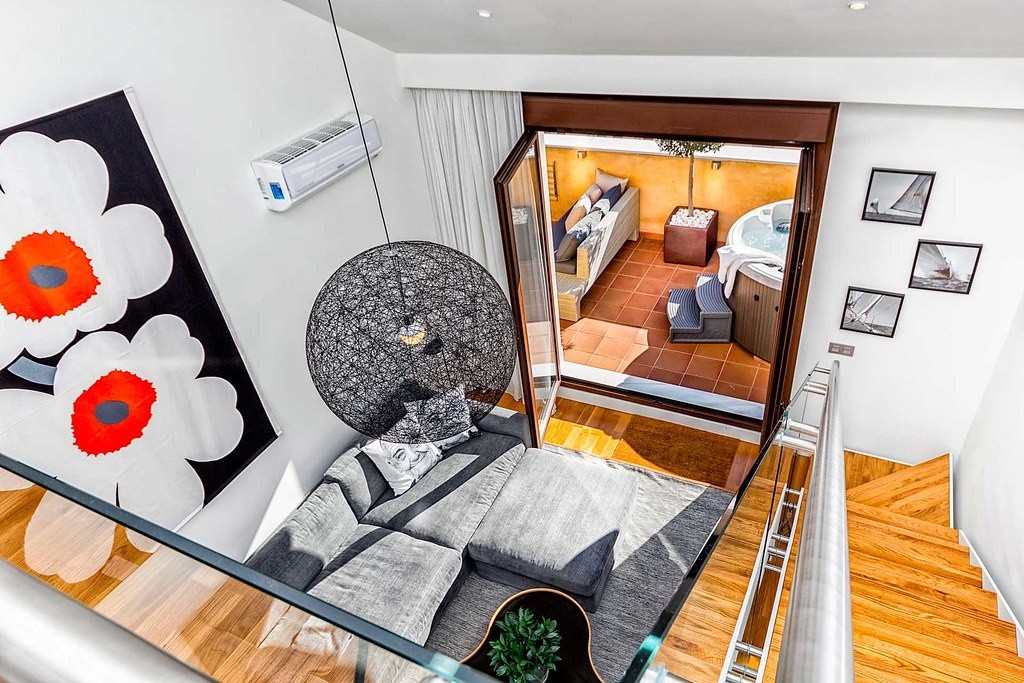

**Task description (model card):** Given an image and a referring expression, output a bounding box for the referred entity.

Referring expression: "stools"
[467,448,638,614]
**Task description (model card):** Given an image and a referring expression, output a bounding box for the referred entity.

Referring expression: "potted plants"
[654,139,724,268]
[486,607,562,683]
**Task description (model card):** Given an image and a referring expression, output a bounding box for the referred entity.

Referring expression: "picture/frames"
[839,285,905,338]
[907,239,983,295]
[861,166,936,226]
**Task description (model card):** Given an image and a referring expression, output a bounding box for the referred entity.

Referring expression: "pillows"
[590,199,610,218]
[403,383,484,452]
[600,182,622,210]
[571,193,592,214]
[564,206,587,233]
[585,183,602,206]
[358,413,444,497]
[555,210,603,263]
[595,168,629,194]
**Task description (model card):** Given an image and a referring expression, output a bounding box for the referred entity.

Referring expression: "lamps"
[305,0,518,445]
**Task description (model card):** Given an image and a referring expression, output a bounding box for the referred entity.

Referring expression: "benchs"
[556,185,640,323]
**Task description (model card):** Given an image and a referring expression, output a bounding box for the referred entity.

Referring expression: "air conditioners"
[250,112,384,213]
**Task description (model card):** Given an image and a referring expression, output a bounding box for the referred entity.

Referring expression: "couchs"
[236,378,532,683]
